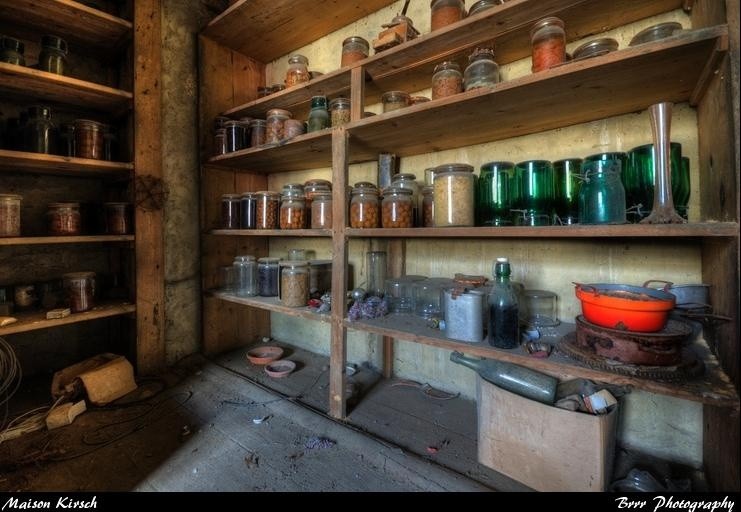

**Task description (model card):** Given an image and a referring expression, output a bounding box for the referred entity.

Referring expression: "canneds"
[341,37,369,68]
[61,272,96,313]
[285,55,311,88]
[310,262,331,297]
[280,261,308,307]
[48,202,80,235]
[328,98,350,127]
[350,163,474,227]
[215,109,304,155]
[62,118,116,162]
[220,179,333,229]
[530,17,565,72]
[383,48,499,115]
[430,0,465,31]
[105,201,130,235]
[258,257,279,296]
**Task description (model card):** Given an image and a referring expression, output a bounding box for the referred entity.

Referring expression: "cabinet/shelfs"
[0,0,138,444]
[198,0,741,492]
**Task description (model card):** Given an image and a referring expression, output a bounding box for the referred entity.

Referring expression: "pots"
[569,278,736,367]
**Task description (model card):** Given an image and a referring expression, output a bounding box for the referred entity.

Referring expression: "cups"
[521,289,560,326]
[380,267,485,344]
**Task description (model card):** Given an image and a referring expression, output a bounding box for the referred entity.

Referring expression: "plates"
[243,342,297,377]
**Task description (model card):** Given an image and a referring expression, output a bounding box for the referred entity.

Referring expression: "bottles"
[212,0,686,155]
[485,260,520,348]
[366,249,387,300]
[444,347,558,405]
[106,201,126,234]
[231,250,330,308]
[36,33,69,76]
[59,269,95,314]
[557,377,636,403]
[62,117,111,162]
[220,164,475,230]
[44,200,80,237]
[474,141,693,228]
[0,33,25,67]
[20,101,59,157]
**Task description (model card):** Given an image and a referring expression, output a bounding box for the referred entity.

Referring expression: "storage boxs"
[473,363,623,492]
